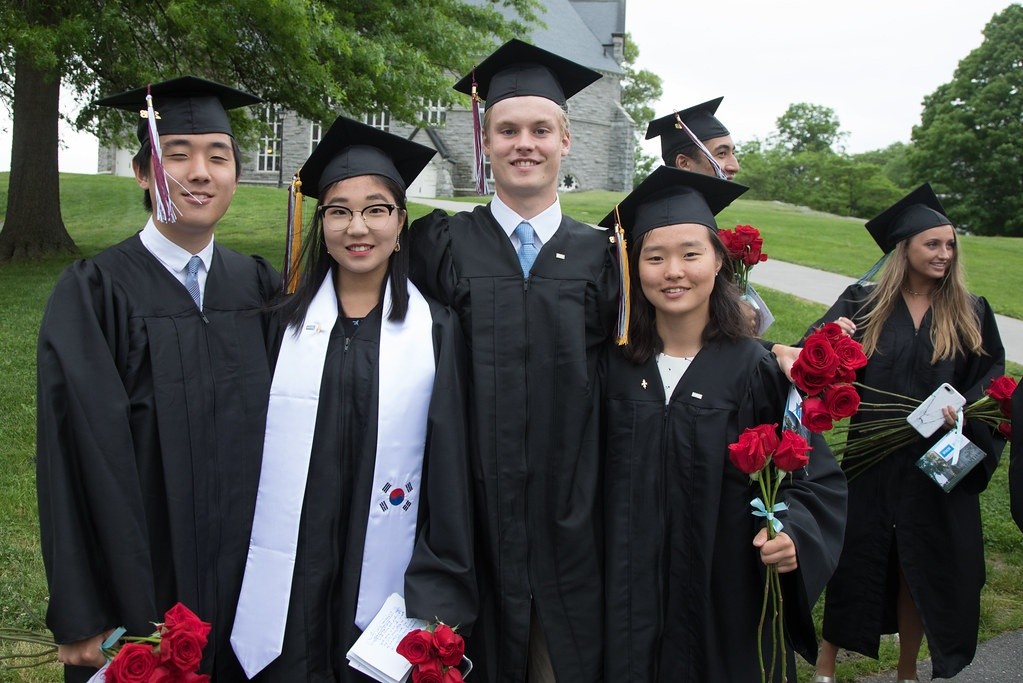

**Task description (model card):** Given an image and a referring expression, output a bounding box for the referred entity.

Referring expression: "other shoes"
[810,669,836,683]
[898,674,918,683]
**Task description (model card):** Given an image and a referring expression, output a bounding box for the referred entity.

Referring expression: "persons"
[1008,376,1023,531]
[597,166,846,683]
[646,98,755,338]
[410,38,803,683]
[790,181,1008,683]
[35,78,283,683]
[208,117,488,683]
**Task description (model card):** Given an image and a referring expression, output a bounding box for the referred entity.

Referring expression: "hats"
[281,115,438,295]
[598,165,750,347]
[645,96,731,181]
[856,183,952,289]
[94,76,265,224]
[453,38,603,195]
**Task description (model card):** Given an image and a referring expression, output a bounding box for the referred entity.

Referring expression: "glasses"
[318,203,401,231]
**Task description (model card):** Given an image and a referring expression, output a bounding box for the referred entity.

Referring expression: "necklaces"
[902,283,929,294]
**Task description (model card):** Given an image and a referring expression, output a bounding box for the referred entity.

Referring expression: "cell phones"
[907,383,967,438]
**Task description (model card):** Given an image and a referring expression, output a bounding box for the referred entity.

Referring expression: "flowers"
[87,604,210,683]
[717,225,772,292]
[727,322,1019,683]
[396,616,467,683]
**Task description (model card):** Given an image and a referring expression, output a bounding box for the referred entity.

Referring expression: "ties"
[184,256,203,309]
[514,220,539,279]
